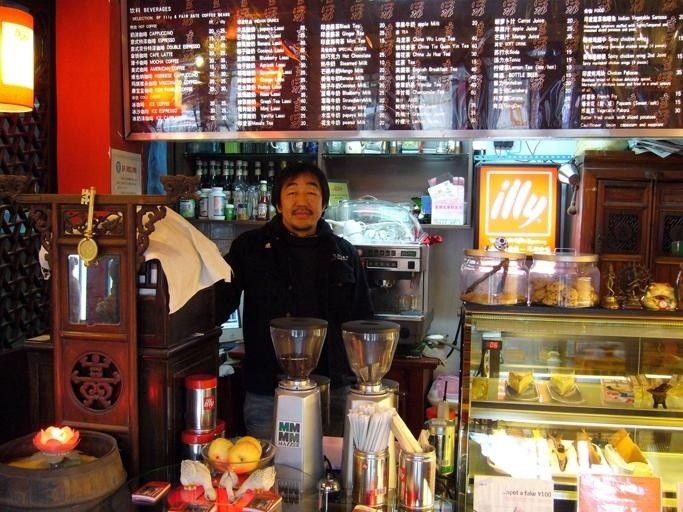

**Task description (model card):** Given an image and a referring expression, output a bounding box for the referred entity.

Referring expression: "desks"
[225,343,440,442]
[88,462,453,512]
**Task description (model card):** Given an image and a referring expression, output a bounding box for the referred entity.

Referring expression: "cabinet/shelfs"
[0,172,221,476]
[570,150,683,374]
[454,300,683,512]
[174,140,473,229]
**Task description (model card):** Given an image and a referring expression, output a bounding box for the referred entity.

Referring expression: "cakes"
[471,377,487,400]
[511,427,654,476]
[507,370,534,392]
[606,384,635,406]
[550,375,576,395]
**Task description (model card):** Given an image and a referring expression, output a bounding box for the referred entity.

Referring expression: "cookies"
[460,292,517,305]
[532,279,600,308]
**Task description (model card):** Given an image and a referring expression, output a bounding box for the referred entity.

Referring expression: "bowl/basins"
[199,436,279,477]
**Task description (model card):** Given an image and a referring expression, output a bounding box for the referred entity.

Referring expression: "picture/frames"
[119,0,683,142]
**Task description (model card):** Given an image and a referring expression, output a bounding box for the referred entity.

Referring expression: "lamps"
[142,62,181,118]
[0,5,35,114]
[252,62,282,96]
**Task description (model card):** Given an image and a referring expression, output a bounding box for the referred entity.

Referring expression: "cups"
[555,164,581,185]
[182,141,367,154]
[398,445,437,510]
[422,418,457,477]
[351,445,391,508]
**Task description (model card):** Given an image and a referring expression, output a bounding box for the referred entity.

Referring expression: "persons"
[213,161,373,445]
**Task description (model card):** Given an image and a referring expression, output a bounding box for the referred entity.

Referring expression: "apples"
[237,435,262,456]
[207,437,234,471]
[227,442,260,472]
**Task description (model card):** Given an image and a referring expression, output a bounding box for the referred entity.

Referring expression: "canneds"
[528,247,601,309]
[460,243,529,306]
[180,196,196,219]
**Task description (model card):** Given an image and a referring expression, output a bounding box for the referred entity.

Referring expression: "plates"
[544,381,586,404]
[504,378,541,404]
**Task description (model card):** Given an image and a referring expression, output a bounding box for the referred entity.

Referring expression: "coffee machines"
[267,313,332,497]
[337,319,403,499]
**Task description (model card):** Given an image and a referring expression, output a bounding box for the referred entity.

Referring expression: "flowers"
[31,425,82,456]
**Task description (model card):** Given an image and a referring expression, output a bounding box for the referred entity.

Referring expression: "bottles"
[546,345,560,374]
[179,156,302,223]
[459,246,682,311]
[177,371,228,476]
[313,465,343,512]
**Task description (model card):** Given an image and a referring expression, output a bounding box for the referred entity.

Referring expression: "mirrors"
[66,253,122,326]
